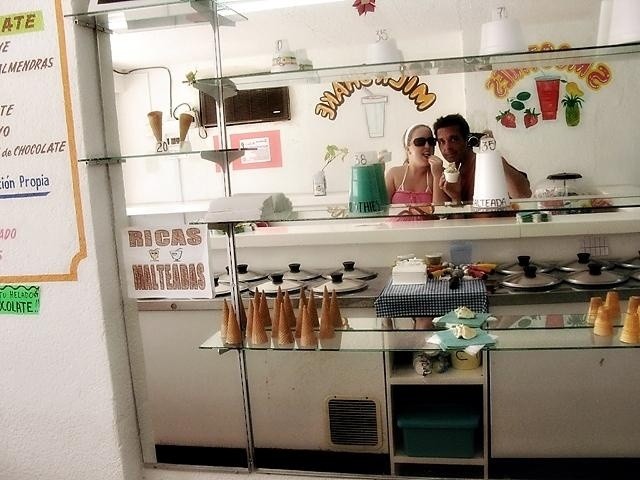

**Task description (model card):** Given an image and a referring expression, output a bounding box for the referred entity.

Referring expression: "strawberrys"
[523,107,541,128]
[495,108,516,129]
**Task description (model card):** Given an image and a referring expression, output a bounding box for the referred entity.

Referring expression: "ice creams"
[443,161,460,183]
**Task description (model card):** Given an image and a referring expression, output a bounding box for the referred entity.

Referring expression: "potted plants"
[312,144,349,196]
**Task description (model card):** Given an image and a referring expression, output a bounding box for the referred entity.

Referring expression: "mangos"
[561,79,584,98]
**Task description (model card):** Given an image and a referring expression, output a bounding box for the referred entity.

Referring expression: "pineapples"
[565,103,580,126]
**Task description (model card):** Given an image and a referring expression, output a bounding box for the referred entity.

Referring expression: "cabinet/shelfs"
[384,350,488,474]
[195,40,638,480]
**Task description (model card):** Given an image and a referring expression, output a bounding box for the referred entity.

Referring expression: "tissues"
[391,257,429,286]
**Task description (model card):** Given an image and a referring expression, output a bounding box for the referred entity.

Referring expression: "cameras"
[465,132,487,148]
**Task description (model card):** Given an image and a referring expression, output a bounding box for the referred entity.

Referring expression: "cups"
[444,167,460,184]
[535,73,561,122]
[424,251,445,275]
[347,160,392,215]
[361,94,388,138]
[451,237,473,272]
[468,138,513,211]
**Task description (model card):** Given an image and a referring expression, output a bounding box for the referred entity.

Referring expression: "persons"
[380,124,448,210]
[433,114,534,200]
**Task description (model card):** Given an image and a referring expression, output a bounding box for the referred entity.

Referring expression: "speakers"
[198,85,290,129]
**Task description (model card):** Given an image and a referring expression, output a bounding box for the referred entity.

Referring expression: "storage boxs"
[396,411,480,459]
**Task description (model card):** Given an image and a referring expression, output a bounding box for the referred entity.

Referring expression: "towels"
[434,325,497,352]
[438,308,493,328]
[374,272,488,317]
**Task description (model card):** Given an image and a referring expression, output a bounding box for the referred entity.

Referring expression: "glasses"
[408,137,437,148]
